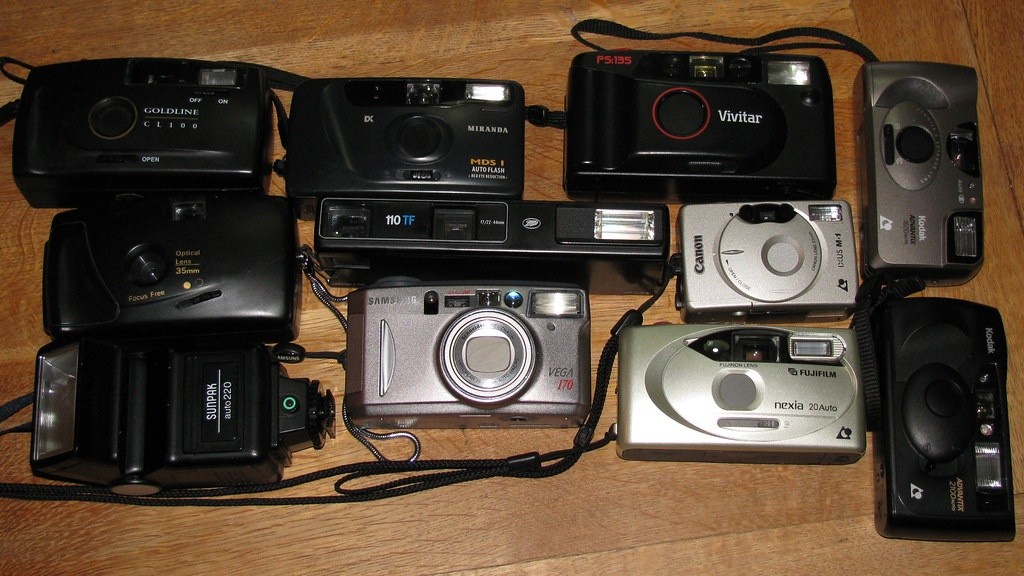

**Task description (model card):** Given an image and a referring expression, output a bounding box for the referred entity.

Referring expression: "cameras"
[12,48,1017,543]
